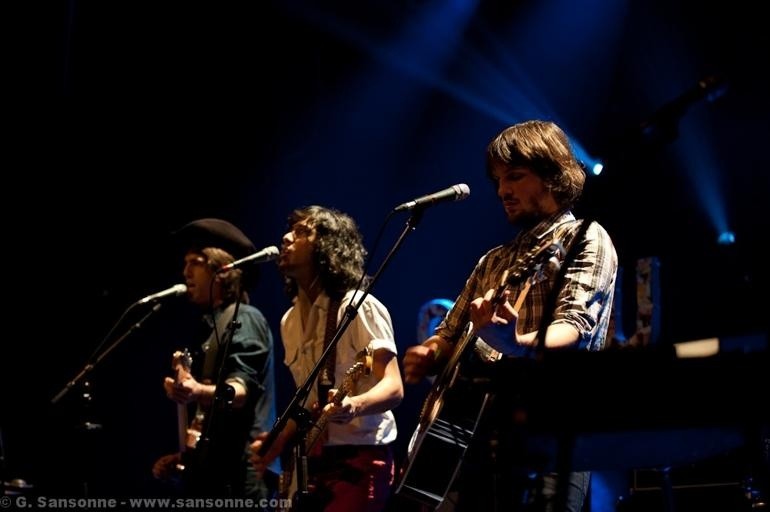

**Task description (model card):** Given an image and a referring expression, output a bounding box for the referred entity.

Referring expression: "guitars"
[169,349,214,472]
[390,239,554,512]
[267,348,374,508]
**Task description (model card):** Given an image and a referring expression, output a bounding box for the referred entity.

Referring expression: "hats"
[164,218,257,272]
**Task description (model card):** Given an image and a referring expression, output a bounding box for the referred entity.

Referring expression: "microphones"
[216,246,280,274]
[395,184,470,213]
[137,283,187,305]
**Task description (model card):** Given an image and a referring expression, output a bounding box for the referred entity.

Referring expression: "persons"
[144,220,283,512]
[243,203,406,511]
[398,117,620,512]
